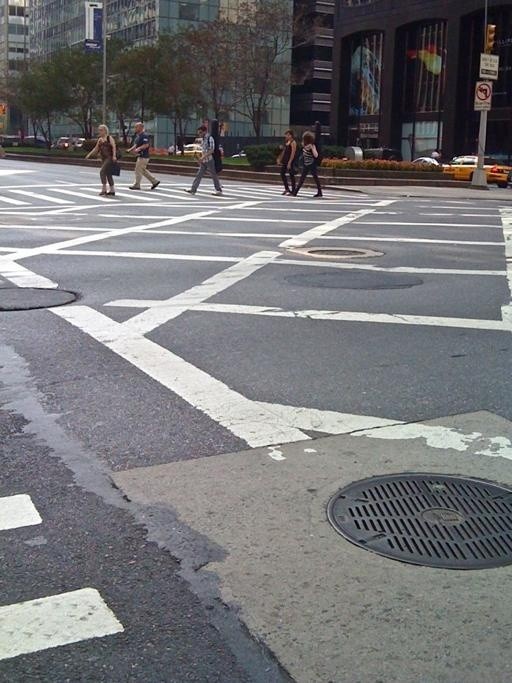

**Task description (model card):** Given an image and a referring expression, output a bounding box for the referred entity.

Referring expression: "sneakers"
[129,187,140,189]
[184,189,195,195]
[151,181,160,188]
[212,191,223,195]
[282,189,322,197]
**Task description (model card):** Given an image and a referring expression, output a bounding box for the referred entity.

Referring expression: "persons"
[287,130,323,197]
[125,121,161,190]
[167,143,184,157]
[276,129,300,196]
[182,124,224,195]
[84,123,117,196]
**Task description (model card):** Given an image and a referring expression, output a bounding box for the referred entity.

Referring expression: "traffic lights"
[486,23,497,51]
[0,104,7,114]
[219,122,228,136]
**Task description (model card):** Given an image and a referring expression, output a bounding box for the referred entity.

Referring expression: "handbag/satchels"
[290,158,299,175]
[212,148,222,173]
[314,156,323,165]
[111,162,120,175]
[110,146,121,159]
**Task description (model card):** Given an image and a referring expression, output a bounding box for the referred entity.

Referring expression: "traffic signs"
[480,53,499,79]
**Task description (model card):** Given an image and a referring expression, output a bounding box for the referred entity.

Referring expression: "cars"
[362,147,511,188]
[1,133,248,158]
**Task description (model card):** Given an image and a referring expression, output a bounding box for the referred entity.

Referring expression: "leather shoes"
[99,191,115,195]
